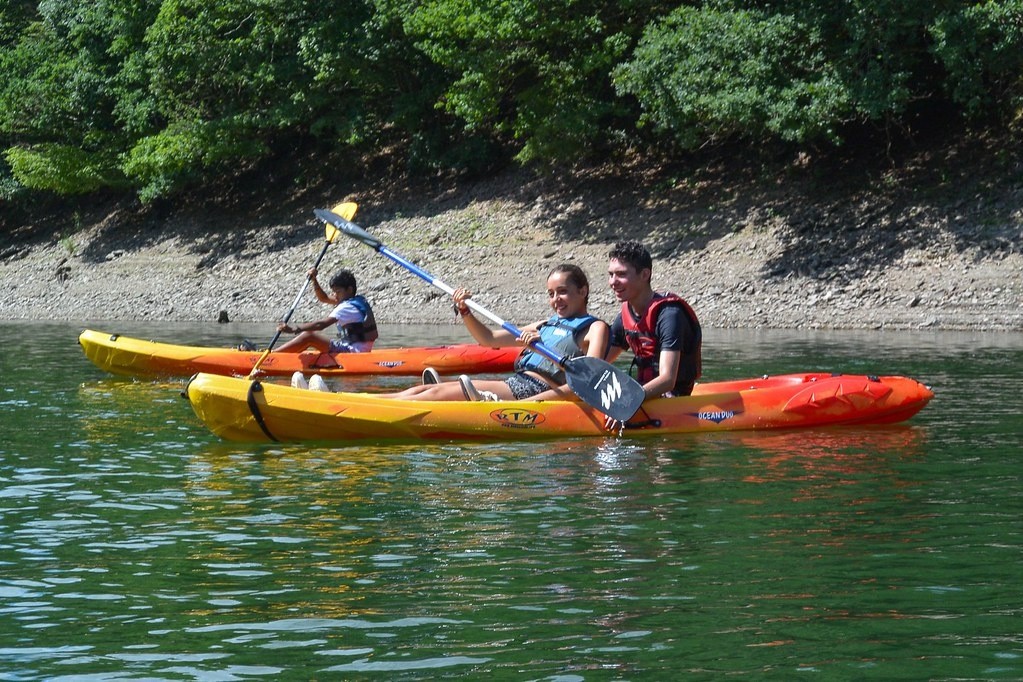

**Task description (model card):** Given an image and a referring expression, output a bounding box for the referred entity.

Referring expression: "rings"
[531,334,533,336]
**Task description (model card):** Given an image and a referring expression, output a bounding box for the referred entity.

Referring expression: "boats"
[79,330,527,377]
[183,368,936,442]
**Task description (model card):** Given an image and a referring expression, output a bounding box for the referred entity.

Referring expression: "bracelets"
[294,326,300,335]
[459,307,470,316]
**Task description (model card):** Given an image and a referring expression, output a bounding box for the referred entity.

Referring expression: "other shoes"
[290,371,309,389]
[459,375,499,402]
[237,338,255,351]
[422,368,441,385]
[308,374,329,392]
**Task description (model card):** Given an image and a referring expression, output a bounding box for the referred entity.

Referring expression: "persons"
[290,264,609,401]
[237,268,378,354]
[421,242,702,430]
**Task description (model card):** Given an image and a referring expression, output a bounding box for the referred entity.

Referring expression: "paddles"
[310,206,648,425]
[247,201,361,377]
